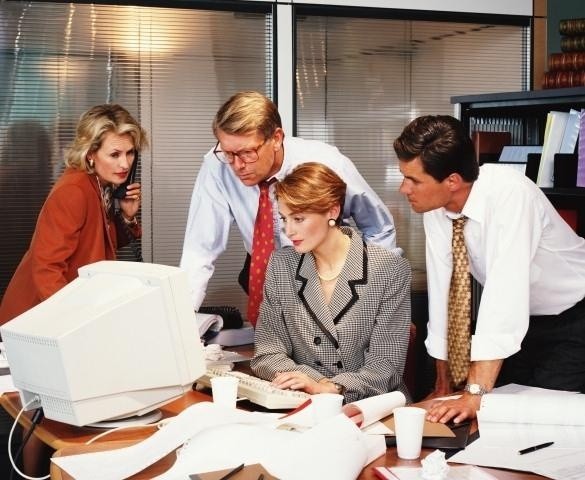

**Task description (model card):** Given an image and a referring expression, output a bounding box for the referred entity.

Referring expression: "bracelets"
[336,384,343,394]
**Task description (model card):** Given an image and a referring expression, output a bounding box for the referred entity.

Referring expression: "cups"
[309,393,346,429]
[392,406,427,460]
[209,376,240,425]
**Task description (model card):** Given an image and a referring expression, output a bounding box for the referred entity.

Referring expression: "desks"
[0,391,551,479]
[223,345,257,376]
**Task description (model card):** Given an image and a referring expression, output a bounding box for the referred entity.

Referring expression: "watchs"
[464,383,488,395]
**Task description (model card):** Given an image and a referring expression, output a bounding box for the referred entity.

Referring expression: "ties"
[447,215,472,387]
[246,181,277,330]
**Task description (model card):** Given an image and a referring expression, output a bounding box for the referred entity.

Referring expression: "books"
[471,107,585,189]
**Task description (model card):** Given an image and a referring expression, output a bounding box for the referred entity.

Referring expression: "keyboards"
[198,367,310,411]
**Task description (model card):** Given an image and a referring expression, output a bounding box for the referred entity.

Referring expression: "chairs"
[405,323,415,399]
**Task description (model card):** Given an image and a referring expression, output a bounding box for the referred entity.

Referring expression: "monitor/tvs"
[0,257,207,428]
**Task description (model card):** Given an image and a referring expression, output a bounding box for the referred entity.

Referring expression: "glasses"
[213,139,267,164]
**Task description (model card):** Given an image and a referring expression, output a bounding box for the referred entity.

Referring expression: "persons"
[250,161,413,410]
[393,114,585,424]
[0,104,147,328]
[178,90,404,340]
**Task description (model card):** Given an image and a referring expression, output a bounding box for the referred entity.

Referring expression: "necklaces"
[315,234,350,282]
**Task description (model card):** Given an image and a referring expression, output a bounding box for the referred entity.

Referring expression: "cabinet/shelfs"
[451,83,584,241]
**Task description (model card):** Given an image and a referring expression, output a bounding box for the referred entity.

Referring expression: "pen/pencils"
[218,464,264,480]
[518,442,554,455]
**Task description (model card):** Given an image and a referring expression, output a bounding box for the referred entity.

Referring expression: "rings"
[137,197,140,201]
